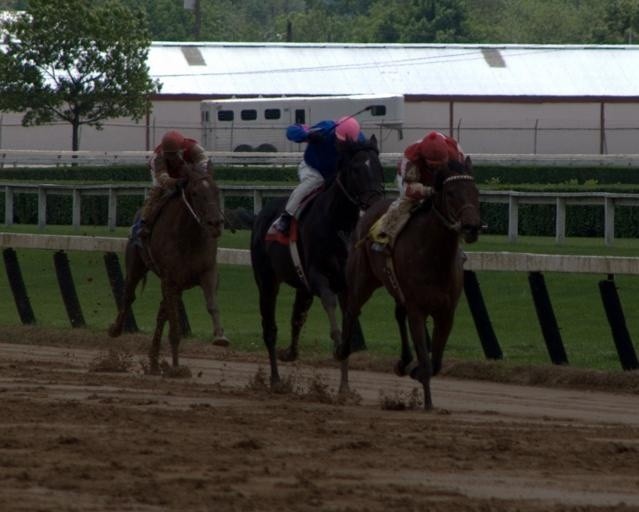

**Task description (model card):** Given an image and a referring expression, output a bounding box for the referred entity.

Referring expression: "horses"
[337,156,484,411]
[108,159,231,376]
[249,133,383,401]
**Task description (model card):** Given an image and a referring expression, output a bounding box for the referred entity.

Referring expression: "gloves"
[309,127,324,145]
[175,177,188,189]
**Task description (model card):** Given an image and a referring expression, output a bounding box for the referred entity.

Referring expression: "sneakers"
[273,210,291,237]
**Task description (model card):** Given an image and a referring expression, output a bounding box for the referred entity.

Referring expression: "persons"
[274,114,366,238]
[131,128,210,249]
[368,131,468,263]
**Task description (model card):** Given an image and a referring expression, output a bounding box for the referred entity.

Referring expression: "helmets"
[420,132,449,166]
[336,116,361,142]
[161,131,185,153]
